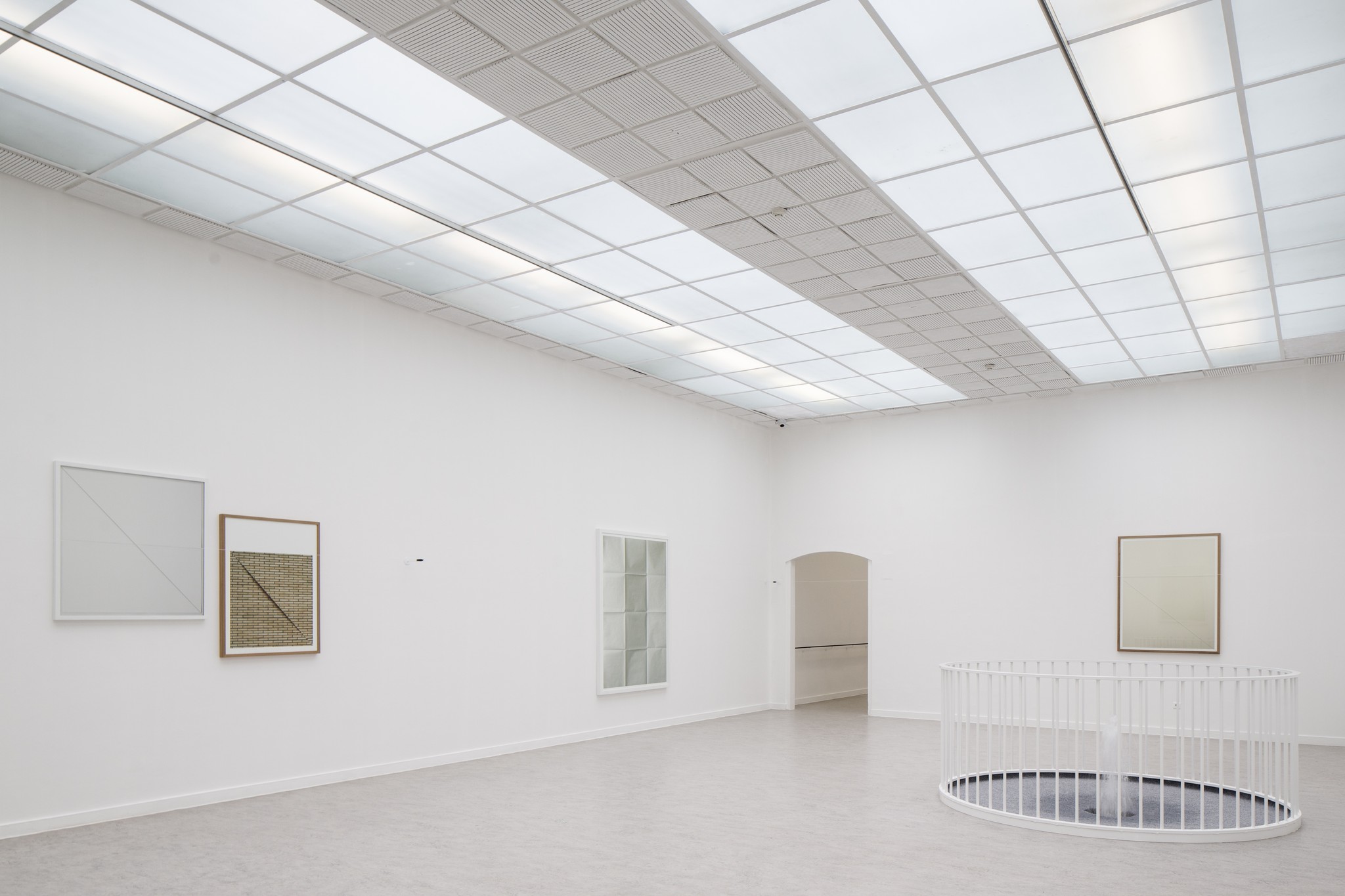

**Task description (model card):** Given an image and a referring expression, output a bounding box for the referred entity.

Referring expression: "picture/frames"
[219,513,320,657]
[1117,533,1220,654]
